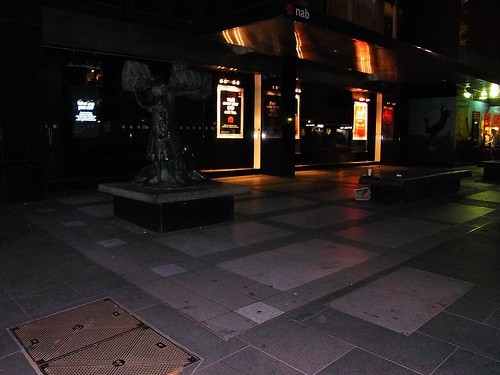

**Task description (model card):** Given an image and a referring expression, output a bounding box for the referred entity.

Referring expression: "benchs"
[478,159,500,180]
[359,167,472,204]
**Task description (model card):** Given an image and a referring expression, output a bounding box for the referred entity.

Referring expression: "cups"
[368,168,372,176]
